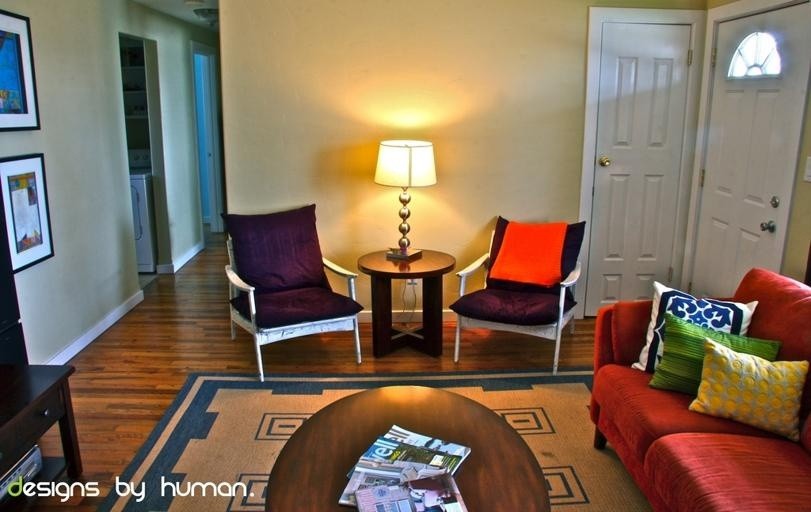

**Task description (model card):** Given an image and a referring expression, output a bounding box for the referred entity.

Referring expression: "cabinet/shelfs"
[0,364,84,512]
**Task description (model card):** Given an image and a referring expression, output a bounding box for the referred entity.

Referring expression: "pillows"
[630,279,809,444]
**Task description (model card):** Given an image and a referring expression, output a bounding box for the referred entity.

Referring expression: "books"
[348,436,461,484]
[384,424,472,475]
[354,473,468,511]
[339,455,448,507]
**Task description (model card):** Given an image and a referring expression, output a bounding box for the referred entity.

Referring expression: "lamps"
[375,140,438,260]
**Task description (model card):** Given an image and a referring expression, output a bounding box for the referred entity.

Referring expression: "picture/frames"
[0,9,41,133]
[0,154,54,272]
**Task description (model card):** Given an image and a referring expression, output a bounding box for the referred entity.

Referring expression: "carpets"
[99,366,656,512]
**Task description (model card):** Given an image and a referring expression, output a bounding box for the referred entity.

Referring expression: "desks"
[358,249,455,357]
[266,383,551,512]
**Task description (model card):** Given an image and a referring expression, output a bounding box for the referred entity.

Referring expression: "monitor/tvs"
[0,174,30,376]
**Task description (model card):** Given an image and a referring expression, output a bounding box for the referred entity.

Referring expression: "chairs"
[455,215,586,375]
[221,203,363,382]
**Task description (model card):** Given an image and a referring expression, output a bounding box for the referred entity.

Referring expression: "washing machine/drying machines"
[129,148,158,273]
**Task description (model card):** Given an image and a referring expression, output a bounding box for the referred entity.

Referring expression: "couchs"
[589,268,811,512]
